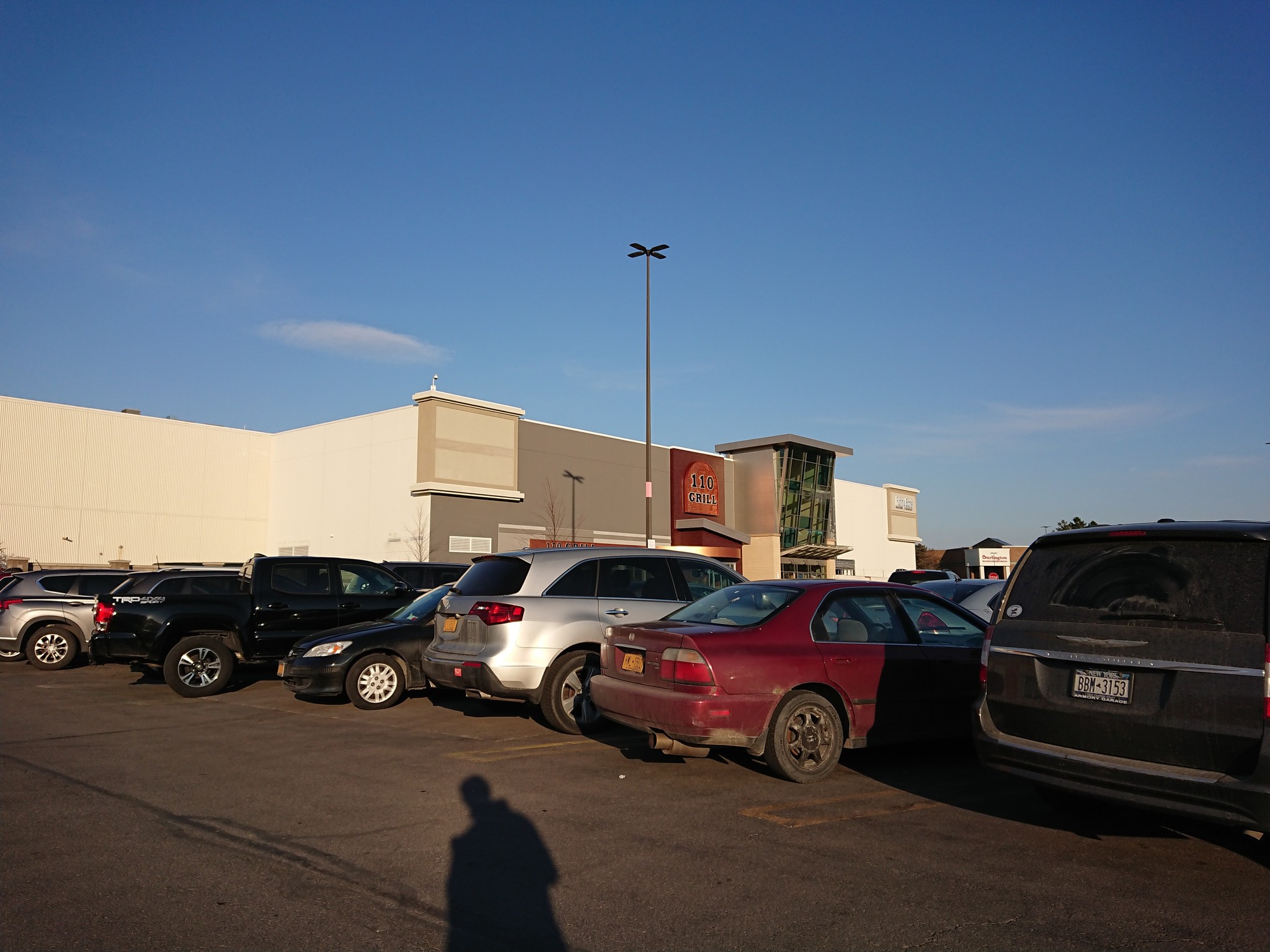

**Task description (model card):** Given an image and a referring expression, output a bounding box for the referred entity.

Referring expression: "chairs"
[837,618,868,642]
[318,572,329,593]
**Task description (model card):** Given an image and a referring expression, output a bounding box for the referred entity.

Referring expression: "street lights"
[627,242,671,548]
[1040,526,1052,534]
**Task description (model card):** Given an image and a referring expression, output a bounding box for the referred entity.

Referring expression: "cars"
[589,579,990,784]
[276,580,459,711]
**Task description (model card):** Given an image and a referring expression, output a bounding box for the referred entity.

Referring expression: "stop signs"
[989,573,999,580]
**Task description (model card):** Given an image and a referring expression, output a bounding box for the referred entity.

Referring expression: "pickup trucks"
[86,552,422,698]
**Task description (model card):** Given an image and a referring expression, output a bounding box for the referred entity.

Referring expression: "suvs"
[970,518,1270,838]
[0,561,241,671]
[421,545,777,735]
[888,569,961,586]
[344,560,471,596]
